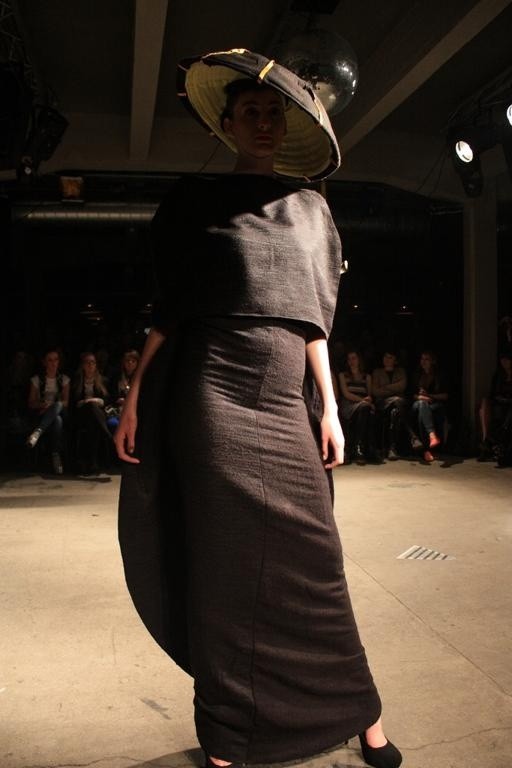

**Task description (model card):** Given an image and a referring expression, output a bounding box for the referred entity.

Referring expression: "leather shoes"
[205,751,243,768]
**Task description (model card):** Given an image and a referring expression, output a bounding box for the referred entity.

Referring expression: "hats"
[186,48,341,187]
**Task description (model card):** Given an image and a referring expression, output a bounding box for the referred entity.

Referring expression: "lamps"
[455,104,500,164]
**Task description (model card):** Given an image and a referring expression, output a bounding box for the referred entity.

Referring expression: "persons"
[111,46,407,767]
[488,349,510,467]
[28,348,143,481]
[305,348,449,460]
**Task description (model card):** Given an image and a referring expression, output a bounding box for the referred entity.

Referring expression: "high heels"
[428,431,441,448]
[387,448,398,461]
[344,730,402,768]
[351,441,364,457]
[422,449,433,461]
[26,431,41,449]
[409,435,425,449]
[49,452,64,474]
